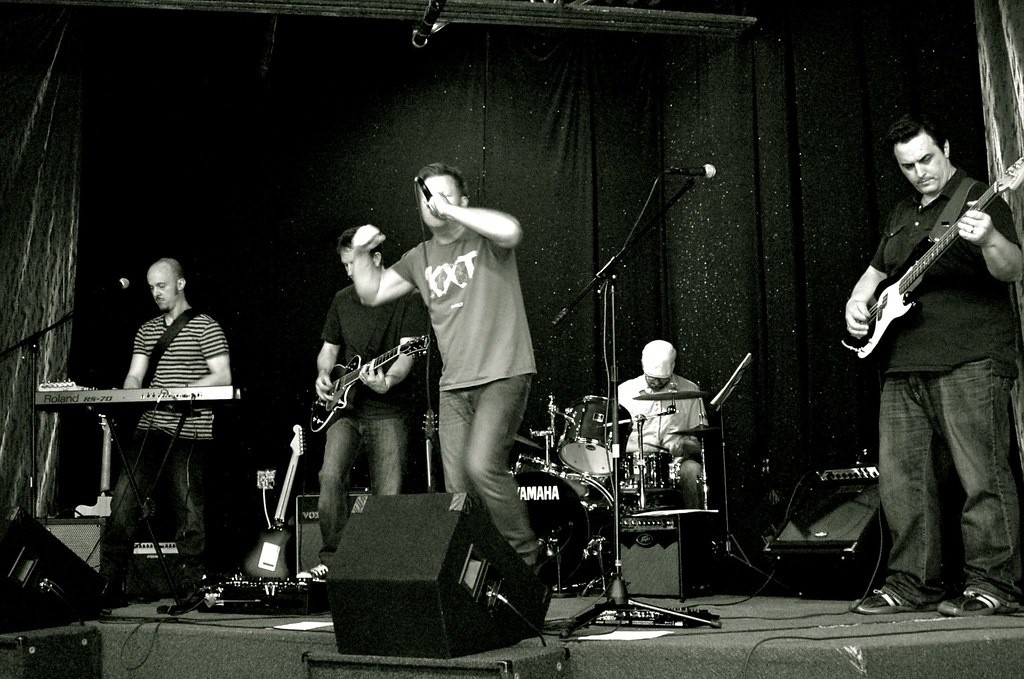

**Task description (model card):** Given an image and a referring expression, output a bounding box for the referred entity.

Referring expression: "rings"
[971,226,974,233]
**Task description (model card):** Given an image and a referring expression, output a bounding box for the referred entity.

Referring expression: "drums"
[561,394,631,454]
[617,450,680,495]
[511,469,618,595]
[556,442,615,485]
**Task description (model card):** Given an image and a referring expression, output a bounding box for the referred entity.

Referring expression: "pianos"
[35,378,245,617]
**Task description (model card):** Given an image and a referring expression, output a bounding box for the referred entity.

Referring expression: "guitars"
[73,414,115,519]
[244,424,307,579]
[424,409,440,493]
[840,155,1024,363]
[310,334,431,433]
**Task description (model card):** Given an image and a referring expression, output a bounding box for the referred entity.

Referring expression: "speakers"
[326,491,552,660]
[764,465,894,601]
[124,540,183,598]
[295,494,373,576]
[37,515,111,573]
[0,505,110,632]
[620,511,727,599]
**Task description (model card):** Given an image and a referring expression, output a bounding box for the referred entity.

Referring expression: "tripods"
[552,176,723,642]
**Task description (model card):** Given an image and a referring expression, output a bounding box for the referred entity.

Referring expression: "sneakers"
[938,586,1019,615]
[850,589,939,614]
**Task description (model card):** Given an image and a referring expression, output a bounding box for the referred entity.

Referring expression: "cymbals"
[668,424,728,437]
[632,390,708,401]
[514,434,543,449]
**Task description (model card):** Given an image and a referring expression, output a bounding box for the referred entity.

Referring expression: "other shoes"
[317,545,338,556]
[105,583,129,602]
[181,584,204,609]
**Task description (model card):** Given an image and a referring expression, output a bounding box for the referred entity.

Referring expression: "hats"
[641,340,676,378]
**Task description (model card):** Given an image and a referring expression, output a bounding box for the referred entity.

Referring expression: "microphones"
[665,164,716,178]
[99,278,130,293]
[416,177,432,202]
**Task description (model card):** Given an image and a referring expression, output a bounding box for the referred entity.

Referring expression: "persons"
[618,340,709,509]
[844,114,1024,616]
[354,162,539,563]
[105,258,232,613]
[299,227,428,582]
